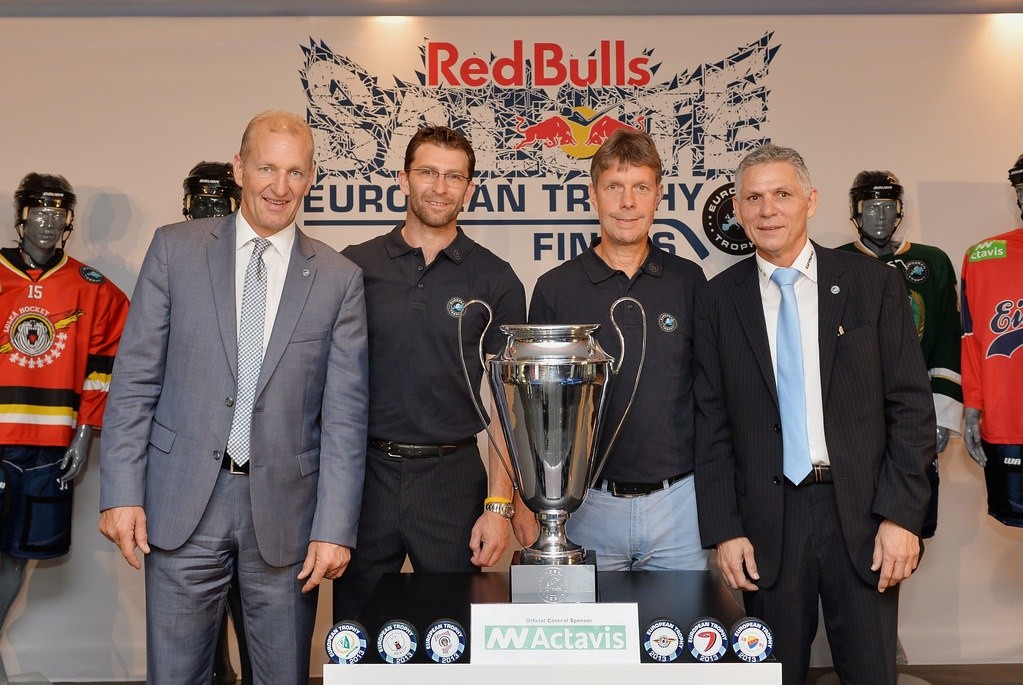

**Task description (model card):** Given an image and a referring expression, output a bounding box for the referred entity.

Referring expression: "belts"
[592,469,694,497]
[367,435,478,459]
[784,464,836,486]
[221,450,252,475]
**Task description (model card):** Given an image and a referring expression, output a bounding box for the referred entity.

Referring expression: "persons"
[0,172,131,685]
[333,125,529,662]
[180,160,243,221]
[834,167,963,455]
[692,145,938,685]
[962,153,1023,530]
[98,111,370,684]
[528,127,712,571]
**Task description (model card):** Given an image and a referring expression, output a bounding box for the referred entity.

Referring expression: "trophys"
[457,297,646,603]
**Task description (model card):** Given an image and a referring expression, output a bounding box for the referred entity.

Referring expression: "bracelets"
[484,496,511,505]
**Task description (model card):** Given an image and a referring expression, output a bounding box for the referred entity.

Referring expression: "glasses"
[404,168,470,189]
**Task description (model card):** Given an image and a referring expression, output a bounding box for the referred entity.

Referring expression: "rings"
[330,571,335,575]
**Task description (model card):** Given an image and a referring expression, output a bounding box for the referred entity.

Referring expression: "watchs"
[486,502,515,521]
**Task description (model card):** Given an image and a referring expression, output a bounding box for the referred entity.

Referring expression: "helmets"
[13,171,78,234]
[182,161,242,213]
[849,168,904,221]
[1008,154,1022,188]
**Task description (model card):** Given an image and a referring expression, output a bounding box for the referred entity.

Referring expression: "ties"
[770,268,814,488]
[225,238,272,467]
